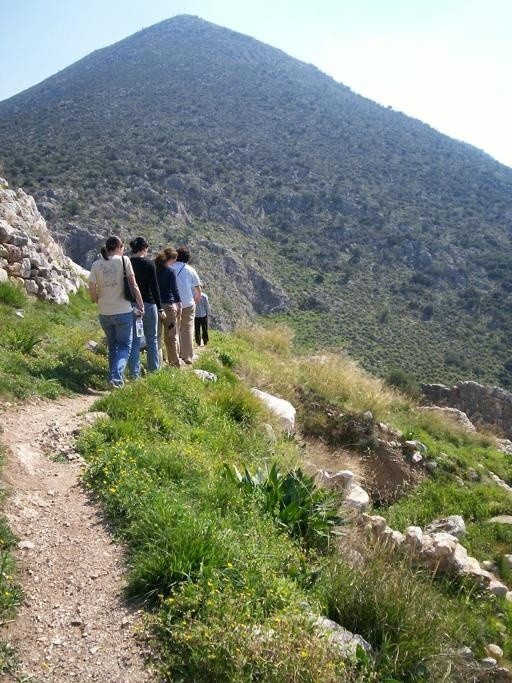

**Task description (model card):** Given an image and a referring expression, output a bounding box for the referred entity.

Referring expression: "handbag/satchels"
[124,277,136,302]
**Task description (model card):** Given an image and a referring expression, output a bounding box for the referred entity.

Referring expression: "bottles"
[136,314,144,338]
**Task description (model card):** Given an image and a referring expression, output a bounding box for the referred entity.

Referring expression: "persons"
[153,246,184,369]
[193,282,211,347]
[124,235,167,374]
[171,245,202,366]
[88,234,145,389]
